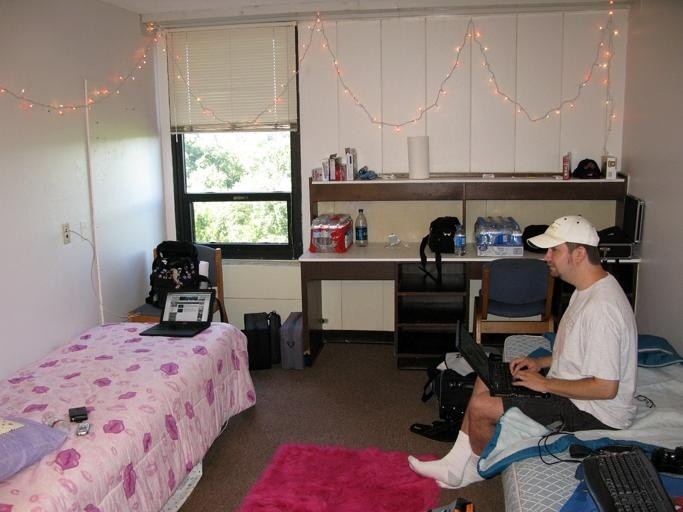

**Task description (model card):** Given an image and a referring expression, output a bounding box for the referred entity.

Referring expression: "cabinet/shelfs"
[296,174,642,365]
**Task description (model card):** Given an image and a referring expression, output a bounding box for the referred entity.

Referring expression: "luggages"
[278,308,307,371]
[240,308,281,372]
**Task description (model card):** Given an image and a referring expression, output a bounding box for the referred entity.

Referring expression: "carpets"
[237,442,444,512]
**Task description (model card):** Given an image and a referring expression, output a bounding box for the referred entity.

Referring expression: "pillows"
[0,414,69,484]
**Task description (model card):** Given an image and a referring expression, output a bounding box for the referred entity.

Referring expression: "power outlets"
[64,223,71,244]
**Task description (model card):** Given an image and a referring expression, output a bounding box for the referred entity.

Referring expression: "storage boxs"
[244,313,273,370]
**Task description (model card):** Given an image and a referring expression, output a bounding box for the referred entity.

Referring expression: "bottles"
[355,209,367,246]
[454,223,465,255]
[472,215,522,247]
[312,213,352,252]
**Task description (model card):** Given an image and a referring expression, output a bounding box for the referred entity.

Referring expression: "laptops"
[139,289,216,337]
[455,320,551,398]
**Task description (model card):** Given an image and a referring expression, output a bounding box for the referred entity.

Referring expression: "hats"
[572,158,602,180]
[524,214,602,252]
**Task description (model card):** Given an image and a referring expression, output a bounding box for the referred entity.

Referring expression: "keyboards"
[582,449,678,512]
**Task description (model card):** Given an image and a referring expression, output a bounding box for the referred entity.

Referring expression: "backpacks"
[145,238,202,309]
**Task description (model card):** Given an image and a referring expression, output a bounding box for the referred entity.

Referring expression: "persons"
[407,214,638,491]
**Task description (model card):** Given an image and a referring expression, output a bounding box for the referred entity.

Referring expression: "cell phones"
[77,423,89,436]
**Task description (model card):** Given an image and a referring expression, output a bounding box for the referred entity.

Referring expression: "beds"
[496,331,683,512]
[0,322,256,512]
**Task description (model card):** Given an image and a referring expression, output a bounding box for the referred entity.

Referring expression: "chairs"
[127,241,229,323]
[476,256,554,348]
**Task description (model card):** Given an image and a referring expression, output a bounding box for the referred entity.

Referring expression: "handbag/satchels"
[429,353,495,425]
[426,216,462,255]
